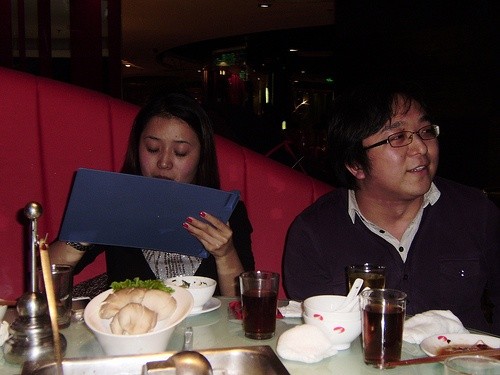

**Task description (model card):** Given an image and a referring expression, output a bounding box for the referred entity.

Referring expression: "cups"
[444,355,500,375]
[359,288,407,369]
[239,270,281,341]
[39,264,74,329]
[346,263,388,296]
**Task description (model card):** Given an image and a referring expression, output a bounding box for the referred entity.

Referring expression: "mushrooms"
[99,287,177,335]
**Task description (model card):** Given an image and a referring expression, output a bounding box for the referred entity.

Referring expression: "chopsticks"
[375,347,500,369]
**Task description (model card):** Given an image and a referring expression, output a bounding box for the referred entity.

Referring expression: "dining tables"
[0,295,500,375]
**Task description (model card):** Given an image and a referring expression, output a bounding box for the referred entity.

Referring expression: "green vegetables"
[110,277,175,295]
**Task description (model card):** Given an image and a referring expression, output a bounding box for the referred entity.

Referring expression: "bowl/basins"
[303,311,361,350]
[0,299,8,324]
[164,276,216,311]
[83,285,194,356]
[303,295,362,321]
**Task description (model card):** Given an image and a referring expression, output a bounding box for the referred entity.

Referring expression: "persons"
[46,92,255,297]
[282,80,500,335]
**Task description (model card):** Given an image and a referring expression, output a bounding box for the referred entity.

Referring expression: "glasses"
[364,124,440,150]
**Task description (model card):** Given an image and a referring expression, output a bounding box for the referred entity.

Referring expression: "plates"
[187,298,222,317]
[419,333,500,365]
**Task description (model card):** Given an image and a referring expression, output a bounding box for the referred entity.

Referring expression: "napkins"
[275,323,335,366]
[398,308,470,347]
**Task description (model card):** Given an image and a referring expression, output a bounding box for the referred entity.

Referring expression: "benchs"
[0,68,341,302]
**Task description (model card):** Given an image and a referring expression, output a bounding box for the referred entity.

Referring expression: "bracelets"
[66,241,90,251]
